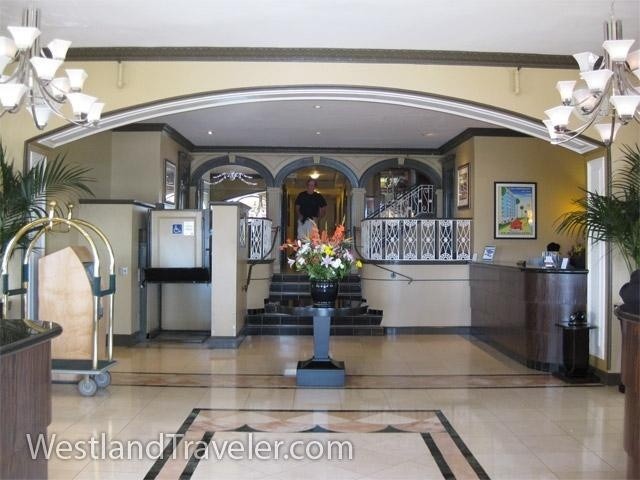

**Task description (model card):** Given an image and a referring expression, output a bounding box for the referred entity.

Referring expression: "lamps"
[0,8,106,133]
[540,20,640,147]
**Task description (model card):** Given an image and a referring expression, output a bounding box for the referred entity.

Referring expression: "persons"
[294,178,326,258]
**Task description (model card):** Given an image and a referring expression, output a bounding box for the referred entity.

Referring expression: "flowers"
[277,223,367,284]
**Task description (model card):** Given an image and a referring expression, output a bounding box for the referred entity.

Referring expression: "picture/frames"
[493,180,538,241]
[456,161,472,210]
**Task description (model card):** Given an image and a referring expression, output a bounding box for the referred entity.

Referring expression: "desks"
[551,321,601,384]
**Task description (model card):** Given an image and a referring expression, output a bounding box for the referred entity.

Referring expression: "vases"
[310,276,338,309]
